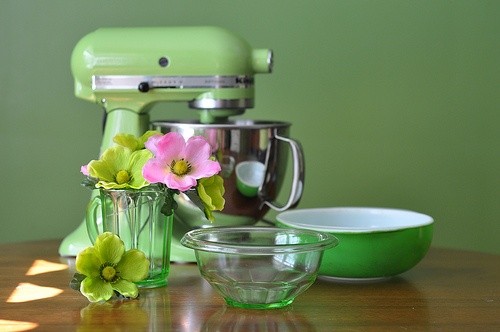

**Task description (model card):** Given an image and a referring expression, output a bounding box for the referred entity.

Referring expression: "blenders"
[57,25,274,262]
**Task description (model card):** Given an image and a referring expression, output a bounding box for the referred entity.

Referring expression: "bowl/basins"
[277,207,434,280]
[181,226,339,308]
[235,160,266,197]
[151,119,305,237]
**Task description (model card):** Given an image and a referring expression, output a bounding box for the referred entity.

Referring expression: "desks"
[0,239,500,332]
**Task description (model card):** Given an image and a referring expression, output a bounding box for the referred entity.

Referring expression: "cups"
[218,154,236,177]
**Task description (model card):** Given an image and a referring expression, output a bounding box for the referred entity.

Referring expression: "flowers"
[69,130,225,303]
